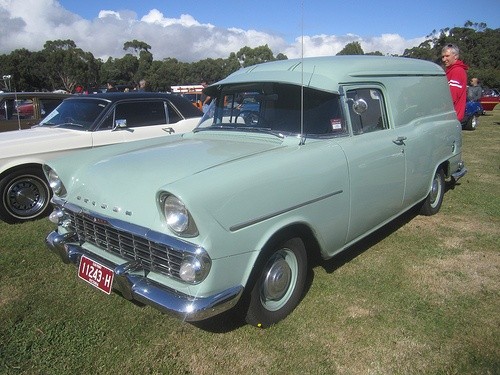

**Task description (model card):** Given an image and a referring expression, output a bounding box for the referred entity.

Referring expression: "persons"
[85,81,102,95]
[139,79,153,92]
[201,78,214,117]
[468,77,482,102]
[106,79,117,93]
[442,43,469,120]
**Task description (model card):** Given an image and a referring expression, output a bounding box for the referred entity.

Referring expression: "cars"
[44,54,467,328]
[0,92,246,225]
[462,86,484,130]
[183,92,228,114]
[0,90,80,133]
